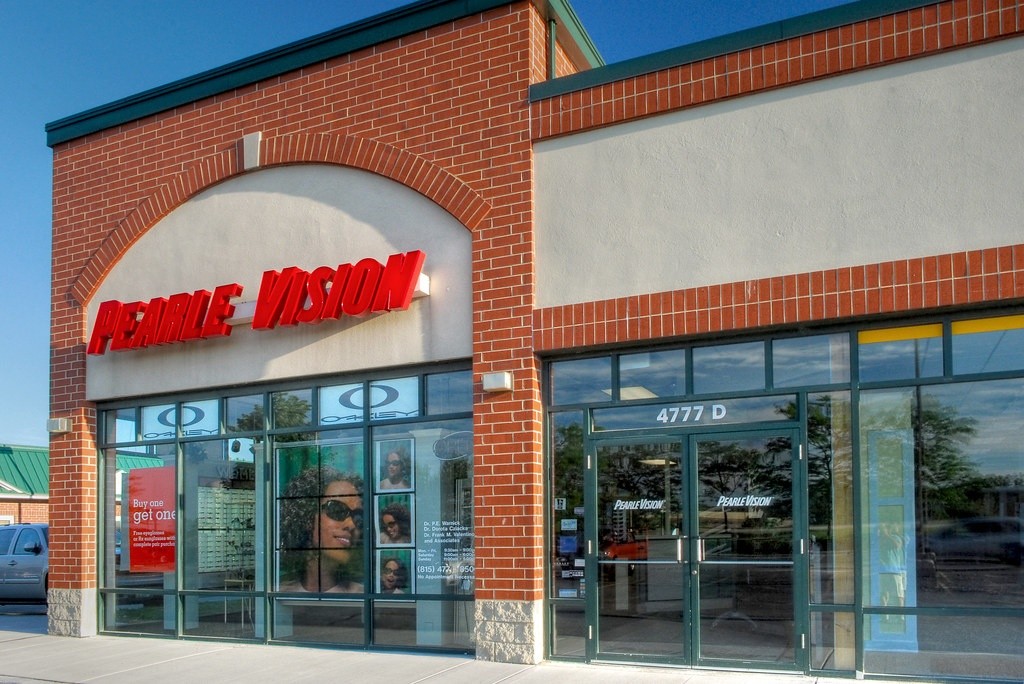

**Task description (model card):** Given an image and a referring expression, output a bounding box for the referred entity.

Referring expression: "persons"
[380,554,408,594]
[379,501,412,545]
[877,519,910,623]
[279,466,365,593]
[379,445,411,490]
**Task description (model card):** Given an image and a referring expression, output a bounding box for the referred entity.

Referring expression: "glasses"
[381,567,401,576]
[384,460,401,467]
[321,500,364,529]
[381,521,396,530]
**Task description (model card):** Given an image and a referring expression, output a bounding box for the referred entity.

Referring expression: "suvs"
[0,522,164,615]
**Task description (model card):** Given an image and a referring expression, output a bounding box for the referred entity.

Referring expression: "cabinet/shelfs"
[224,502,256,629]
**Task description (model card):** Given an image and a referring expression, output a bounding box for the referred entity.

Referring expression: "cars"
[924,517,1024,565]
[560,525,649,581]
